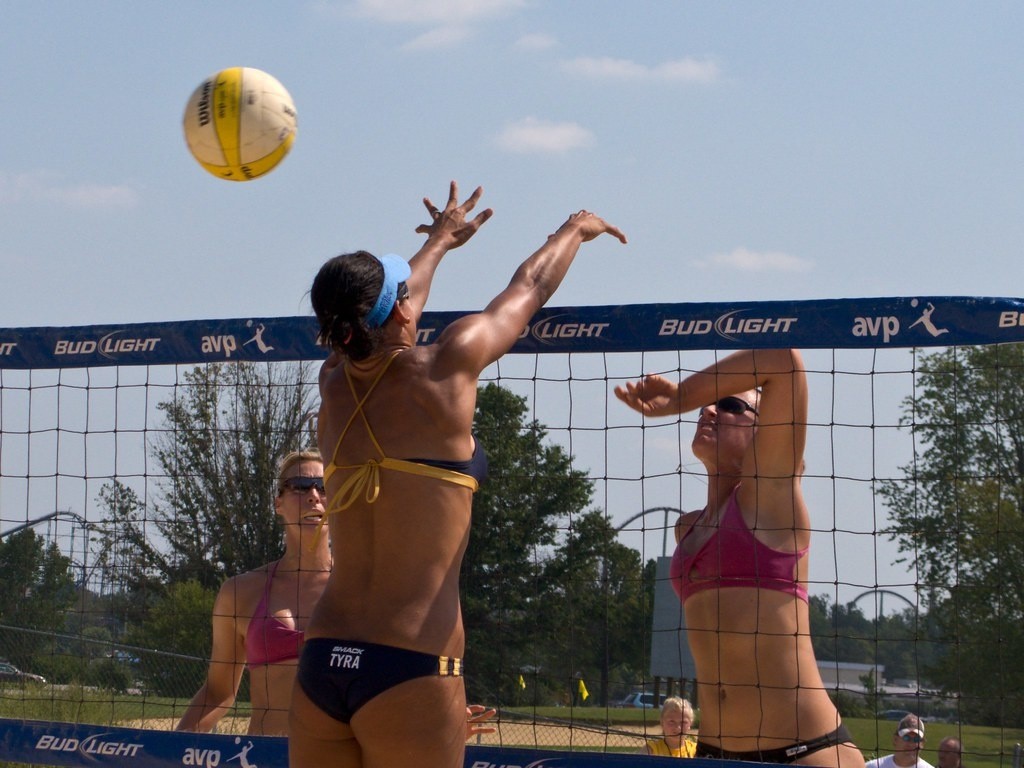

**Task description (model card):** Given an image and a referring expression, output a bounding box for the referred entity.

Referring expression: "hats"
[360,252,412,332]
[898,713,925,738]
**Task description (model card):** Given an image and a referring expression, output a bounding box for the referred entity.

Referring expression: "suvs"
[622,692,672,709]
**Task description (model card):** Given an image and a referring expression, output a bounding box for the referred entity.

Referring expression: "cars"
[598,701,635,708]
[873,710,927,724]
[0,663,47,689]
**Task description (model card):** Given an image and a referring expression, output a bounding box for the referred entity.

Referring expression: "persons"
[287,180,626,768]
[935,736,966,768]
[176,451,497,743]
[614,349,865,768]
[865,713,935,768]
[639,696,697,758]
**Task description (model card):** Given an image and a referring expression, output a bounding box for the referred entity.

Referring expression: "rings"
[432,211,441,215]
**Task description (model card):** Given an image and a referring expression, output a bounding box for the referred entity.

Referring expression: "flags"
[579,680,589,700]
[519,676,526,690]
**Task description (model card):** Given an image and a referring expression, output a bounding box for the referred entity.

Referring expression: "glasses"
[902,734,920,743]
[699,395,759,418]
[278,475,326,498]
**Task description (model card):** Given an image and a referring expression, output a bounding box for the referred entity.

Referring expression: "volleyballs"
[180,65,298,183]
